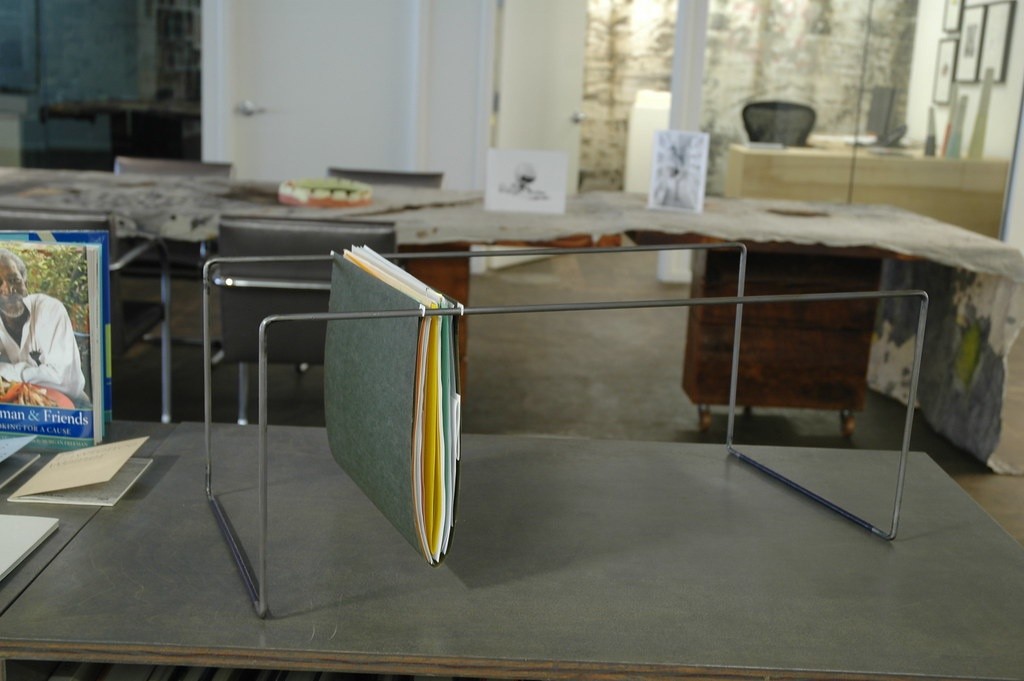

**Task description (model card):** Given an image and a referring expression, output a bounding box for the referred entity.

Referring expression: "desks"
[0,166,1024,478]
[0,422,1024,681]
[0,420,180,621]
[721,144,1008,238]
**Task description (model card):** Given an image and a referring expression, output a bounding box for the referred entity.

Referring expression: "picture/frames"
[977,0,1017,84]
[941,0,965,34]
[952,4,988,84]
[932,37,958,106]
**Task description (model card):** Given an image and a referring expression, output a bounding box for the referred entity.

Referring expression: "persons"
[0,246,91,408]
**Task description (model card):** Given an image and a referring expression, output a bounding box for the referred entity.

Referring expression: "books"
[7,435,155,507]
[1,229,111,489]
[323,243,462,568]
[0,514,61,580]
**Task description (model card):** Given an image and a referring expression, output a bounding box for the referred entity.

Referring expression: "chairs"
[327,168,445,189]
[742,103,816,147]
[114,156,235,178]
[209,213,400,426]
[0,204,172,424]
